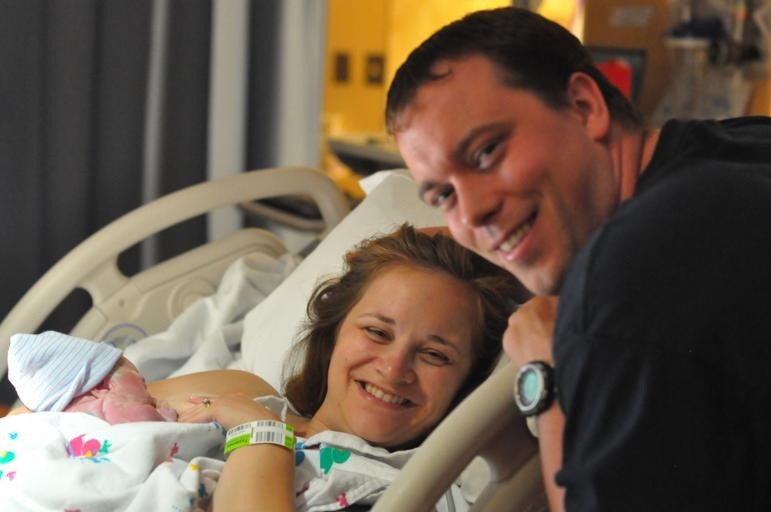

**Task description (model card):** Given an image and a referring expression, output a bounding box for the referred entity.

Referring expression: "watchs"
[513,360,557,416]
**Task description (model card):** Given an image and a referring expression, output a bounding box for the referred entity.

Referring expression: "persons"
[8,331,179,425]
[386,6,771,512]
[0,221,532,512]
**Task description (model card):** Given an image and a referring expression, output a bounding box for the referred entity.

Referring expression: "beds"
[0,168,550,511]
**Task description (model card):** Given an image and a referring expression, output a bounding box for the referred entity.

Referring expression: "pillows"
[239,170,539,506]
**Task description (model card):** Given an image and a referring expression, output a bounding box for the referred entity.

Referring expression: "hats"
[6,330,128,412]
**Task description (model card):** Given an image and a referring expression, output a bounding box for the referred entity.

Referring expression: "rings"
[201,398,210,407]
